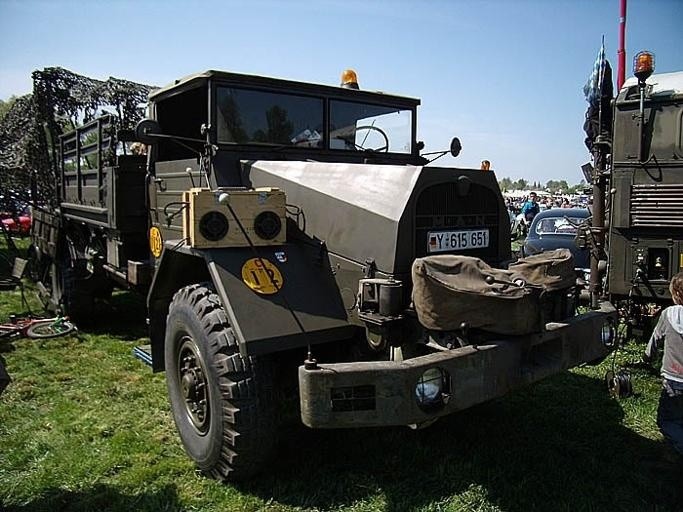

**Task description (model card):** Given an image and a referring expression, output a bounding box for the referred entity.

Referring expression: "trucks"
[580,34,682,350]
[0,67,619,486]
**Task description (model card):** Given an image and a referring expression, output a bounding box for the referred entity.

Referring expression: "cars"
[501,188,609,303]
[0,188,45,238]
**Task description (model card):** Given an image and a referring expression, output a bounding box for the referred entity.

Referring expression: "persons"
[643,270,683,449]
[503,186,591,235]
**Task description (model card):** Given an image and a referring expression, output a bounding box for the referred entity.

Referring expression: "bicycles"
[0,294,73,339]
